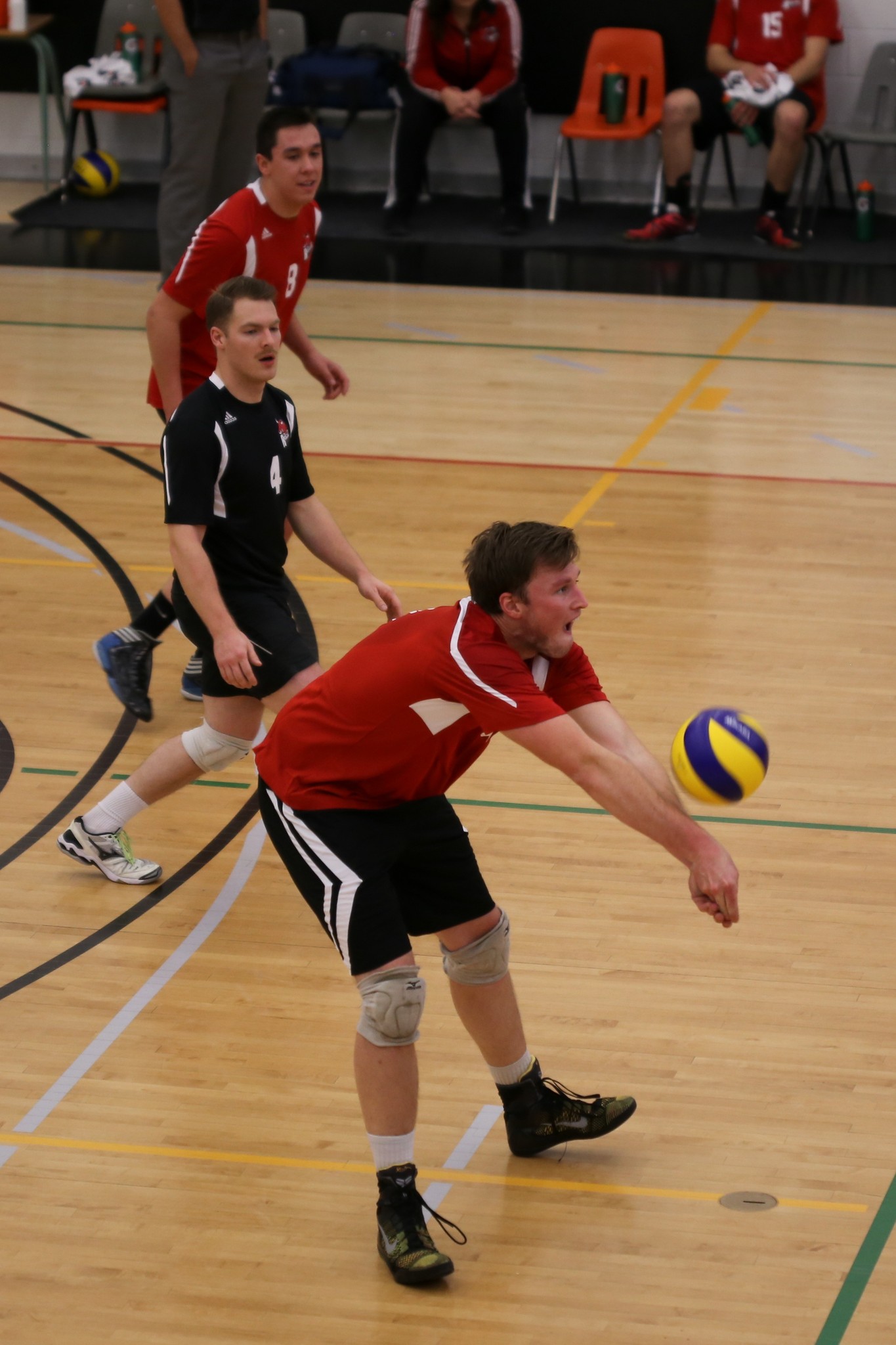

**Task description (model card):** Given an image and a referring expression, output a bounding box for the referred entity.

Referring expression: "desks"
[0,16,59,195]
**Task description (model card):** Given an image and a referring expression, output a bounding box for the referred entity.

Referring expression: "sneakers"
[497,1053,638,1157]
[376,1166,469,1286]
[625,211,696,241]
[95,626,164,724]
[754,207,805,249]
[57,815,163,885]
[179,655,204,701]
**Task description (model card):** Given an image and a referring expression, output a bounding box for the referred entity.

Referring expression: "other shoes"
[379,191,429,235]
[498,208,529,232]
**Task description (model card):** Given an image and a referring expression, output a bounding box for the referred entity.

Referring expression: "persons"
[95,103,350,719]
[255,521,739,1291]
[625,0,844,250]
[158,0,273,291]
[56,296,399,885]
[376,0,543,235]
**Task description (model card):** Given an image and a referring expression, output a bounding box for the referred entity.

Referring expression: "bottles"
[605,63,623,124]
[119,22,140,85]
[854,179,874,240]
[722,92,760,147]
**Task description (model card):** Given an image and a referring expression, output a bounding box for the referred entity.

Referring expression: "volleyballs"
[69,149,120,198]
[670,707,769,807]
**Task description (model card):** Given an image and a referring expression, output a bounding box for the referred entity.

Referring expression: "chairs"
[545,25,669,222]
[692,41,896,240]
[59,0,174,185]
[257,11,412,211]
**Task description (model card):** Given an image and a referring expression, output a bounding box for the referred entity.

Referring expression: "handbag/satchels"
[270,41,407,140]
